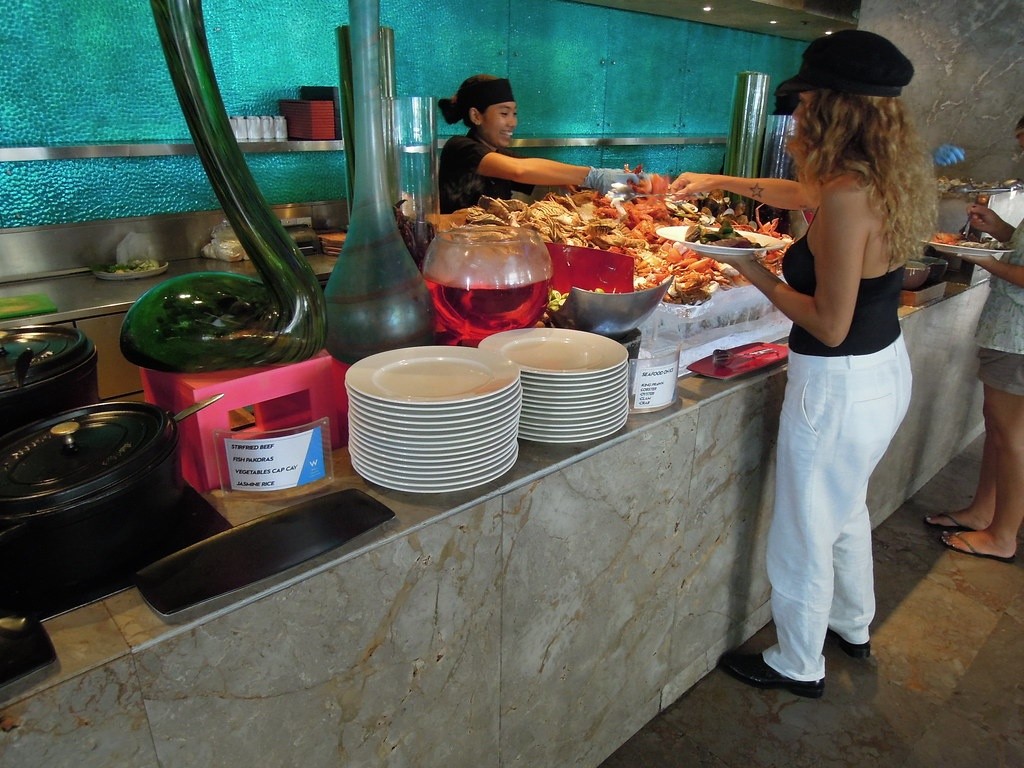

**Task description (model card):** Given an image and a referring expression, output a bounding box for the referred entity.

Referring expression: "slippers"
[924,513,975,531]
[939,530,1016,563]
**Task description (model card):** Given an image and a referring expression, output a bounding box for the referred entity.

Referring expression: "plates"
[920,239,1016,256]
[280,87,342,140]
[92,262,168,280]
[343,328,628,494]
[656,226,786,256]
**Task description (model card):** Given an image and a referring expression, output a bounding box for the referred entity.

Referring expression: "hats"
[777,29,915,97]
[455,73,515,111]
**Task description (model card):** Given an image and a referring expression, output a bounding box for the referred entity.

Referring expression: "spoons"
[959,206,976,240]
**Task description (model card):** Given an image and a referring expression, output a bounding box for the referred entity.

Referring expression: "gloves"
[582,165,639,196]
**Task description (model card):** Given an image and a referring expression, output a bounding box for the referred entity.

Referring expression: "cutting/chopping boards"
[0,293,58,318]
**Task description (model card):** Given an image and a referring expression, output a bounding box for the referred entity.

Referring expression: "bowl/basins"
[902,261,930,289]
[910,256,948,282]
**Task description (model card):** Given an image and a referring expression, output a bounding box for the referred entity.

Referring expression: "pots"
[0,402,181,582]
[0,325,98,437]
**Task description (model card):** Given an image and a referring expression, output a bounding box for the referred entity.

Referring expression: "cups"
[423,227,553,347]
[232,116,250,139]
[274,116,288,138]
[229,119,237,135]
[247,116,261,138]
[260,116,274,138]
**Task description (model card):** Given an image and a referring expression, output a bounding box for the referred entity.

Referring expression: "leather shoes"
[717,649,826,699]
[828,628,871,659]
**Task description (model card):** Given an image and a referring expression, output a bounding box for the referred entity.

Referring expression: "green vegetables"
[109,255,158,273]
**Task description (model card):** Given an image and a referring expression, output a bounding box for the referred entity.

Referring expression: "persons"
[667,27,914,699]
[921,117,1024,566]
[438,73,652,211]
